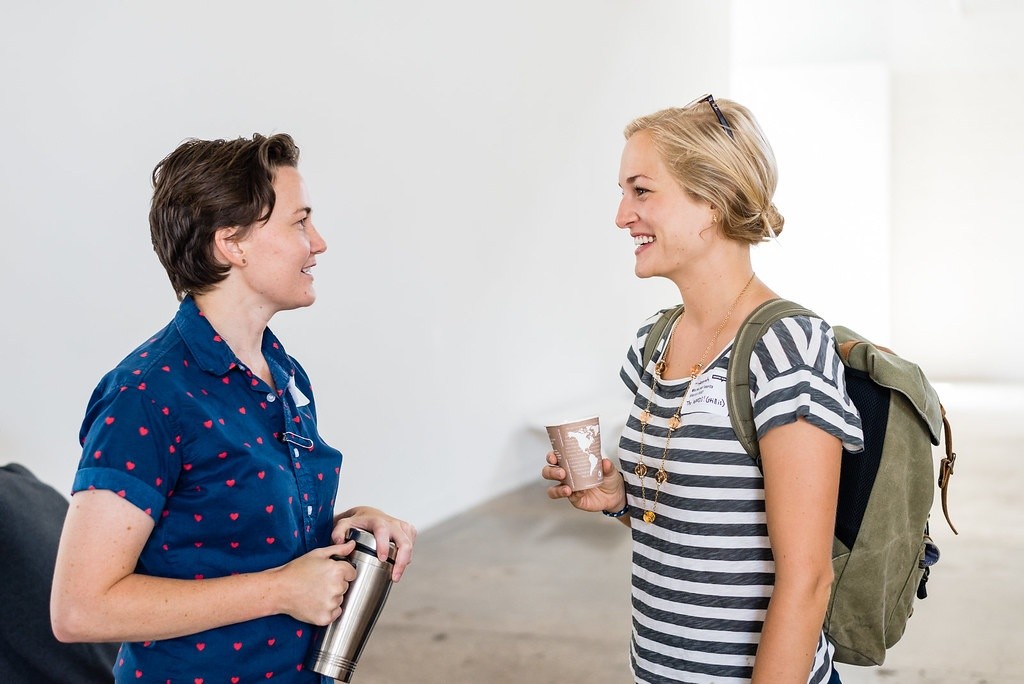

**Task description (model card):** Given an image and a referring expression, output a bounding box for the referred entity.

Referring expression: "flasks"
[304,527,398,683]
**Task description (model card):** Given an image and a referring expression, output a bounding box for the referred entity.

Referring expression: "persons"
[50,133,417,684]
[0,461,122,684]
[542,93,866,684]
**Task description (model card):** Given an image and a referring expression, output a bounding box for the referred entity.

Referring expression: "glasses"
[683,93,734,141]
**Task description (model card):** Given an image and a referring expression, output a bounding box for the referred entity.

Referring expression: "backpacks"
[640,303,955,666]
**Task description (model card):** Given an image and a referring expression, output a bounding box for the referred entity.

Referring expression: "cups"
[545,417,604,491]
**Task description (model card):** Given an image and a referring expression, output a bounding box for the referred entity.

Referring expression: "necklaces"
[602,503,629,517]
[635,271,756,523]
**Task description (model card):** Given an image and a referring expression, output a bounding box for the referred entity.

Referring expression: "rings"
[343,580,349,596]
